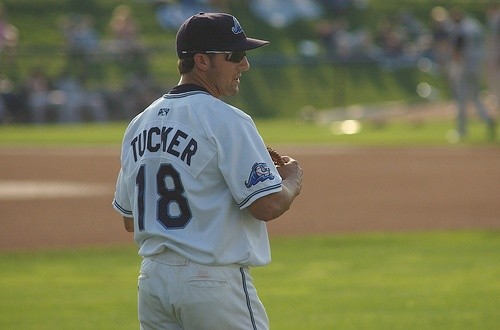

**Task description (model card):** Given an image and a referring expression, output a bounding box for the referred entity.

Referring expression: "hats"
[175,12,270,58]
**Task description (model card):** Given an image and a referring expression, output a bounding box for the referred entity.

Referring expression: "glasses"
[226,51,247,63]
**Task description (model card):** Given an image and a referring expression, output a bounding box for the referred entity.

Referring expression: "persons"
[0,4,500,142]
[111,12,304,330]
